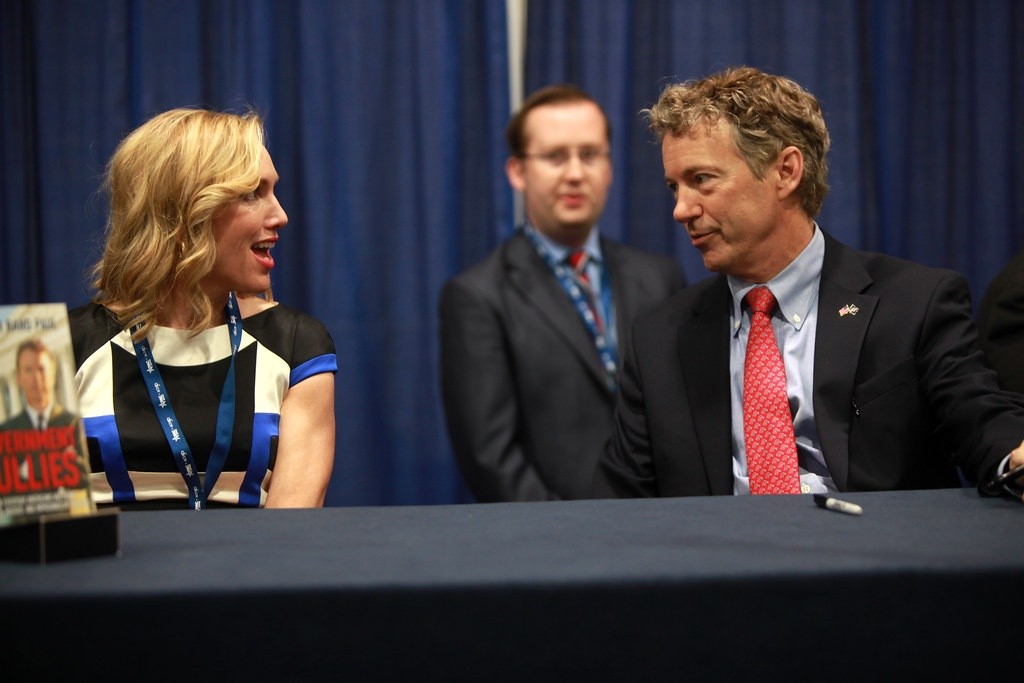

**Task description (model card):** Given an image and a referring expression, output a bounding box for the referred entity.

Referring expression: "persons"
[583,67,1024,503]
[0,340,88,525]
[68,107,339,511]
[438,85,691,505]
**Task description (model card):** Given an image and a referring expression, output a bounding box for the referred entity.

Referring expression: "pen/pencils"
[813,494,863,516]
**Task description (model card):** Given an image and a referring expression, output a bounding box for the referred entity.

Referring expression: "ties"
[746,285,800,494]
[38,413,44,431]
[569,251,603,335]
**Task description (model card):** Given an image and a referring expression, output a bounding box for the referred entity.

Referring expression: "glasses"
[524,143,611,165]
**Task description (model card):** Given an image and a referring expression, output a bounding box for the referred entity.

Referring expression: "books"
[0,304,97,525]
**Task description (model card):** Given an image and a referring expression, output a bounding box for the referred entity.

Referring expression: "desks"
[0,487,1024,683]
